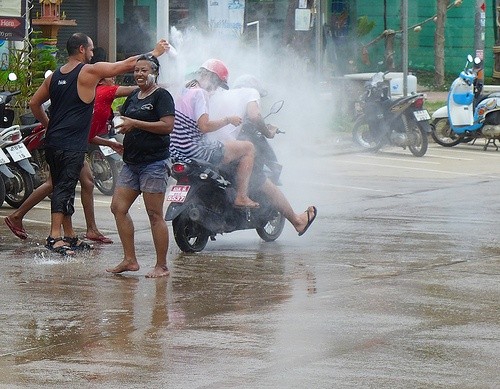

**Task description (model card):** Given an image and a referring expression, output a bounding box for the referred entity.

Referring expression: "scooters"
[430,54,500,152]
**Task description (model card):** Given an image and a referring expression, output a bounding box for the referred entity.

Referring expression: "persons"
[4,33,175,278]
[169,59,317,236]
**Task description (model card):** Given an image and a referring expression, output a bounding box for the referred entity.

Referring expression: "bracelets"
[225,117,230,125]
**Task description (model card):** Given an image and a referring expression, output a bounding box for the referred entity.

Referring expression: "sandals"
[44,236,76,256]
[63,236,90,250]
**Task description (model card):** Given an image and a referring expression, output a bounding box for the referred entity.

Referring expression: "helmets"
[200,59,230,91]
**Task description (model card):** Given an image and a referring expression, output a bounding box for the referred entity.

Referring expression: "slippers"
[85,232,114,244]
[233,201,260,209]
[4,217,28,240]
[298,206,317,236]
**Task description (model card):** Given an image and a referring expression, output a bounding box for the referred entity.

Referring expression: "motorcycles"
[351,69,431,159]
[0,69,121,210]
[165,100,286,252]
